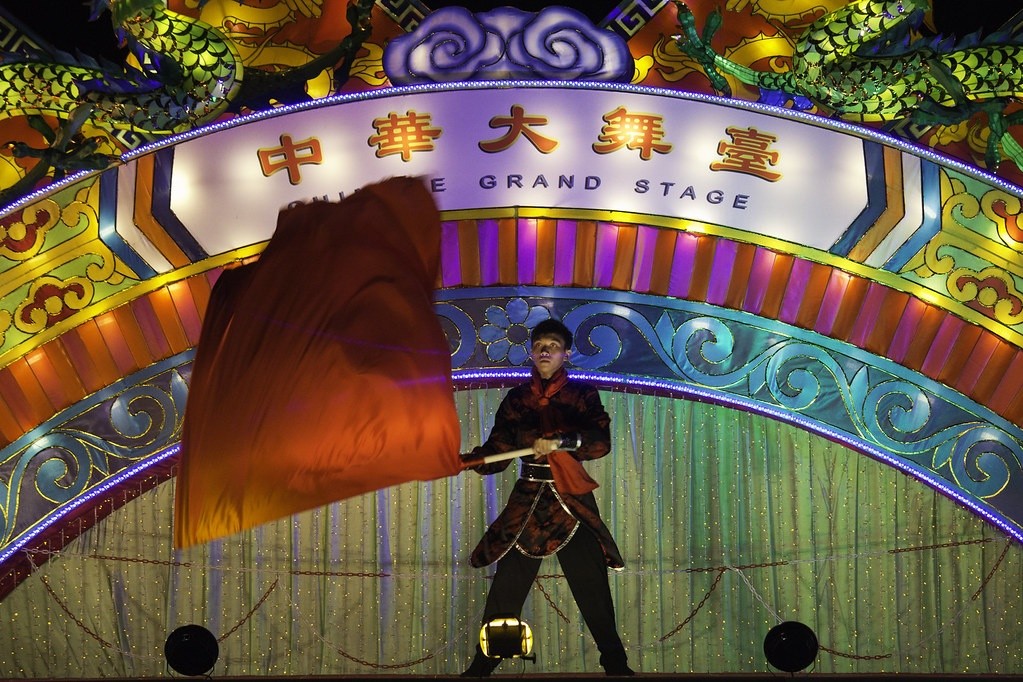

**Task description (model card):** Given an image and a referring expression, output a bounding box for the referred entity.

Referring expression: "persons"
[450,318,636,678]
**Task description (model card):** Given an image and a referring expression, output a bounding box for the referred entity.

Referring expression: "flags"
[172,175,462,550]
